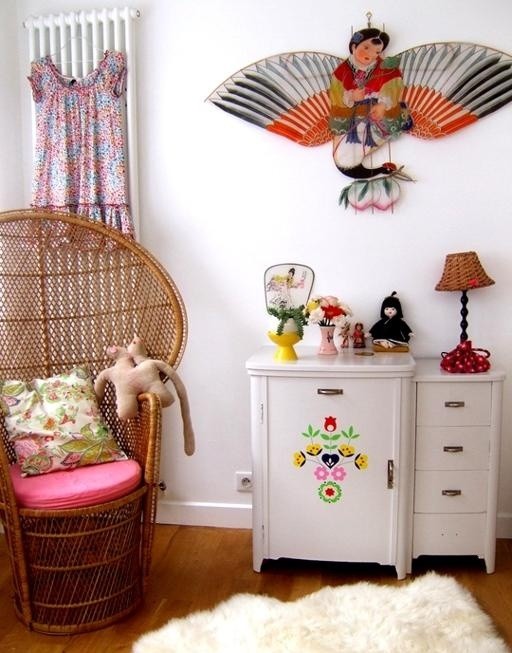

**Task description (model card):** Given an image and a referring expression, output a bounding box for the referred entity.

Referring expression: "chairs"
[0,208,189,636]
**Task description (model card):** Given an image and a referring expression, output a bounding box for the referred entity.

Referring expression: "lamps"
[435,252,495,342]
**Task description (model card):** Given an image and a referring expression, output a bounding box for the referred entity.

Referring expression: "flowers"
[306,295,354,326]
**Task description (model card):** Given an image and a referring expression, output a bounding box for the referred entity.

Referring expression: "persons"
[329,29,402,133]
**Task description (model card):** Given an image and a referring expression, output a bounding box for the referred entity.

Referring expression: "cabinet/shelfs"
[412,354,505,575]
[245,344,417,581]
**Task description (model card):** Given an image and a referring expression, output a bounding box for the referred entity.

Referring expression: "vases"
[267,330,303,362]
[317,326,339,355]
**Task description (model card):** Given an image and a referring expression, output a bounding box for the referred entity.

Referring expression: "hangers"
[47,9,105,66]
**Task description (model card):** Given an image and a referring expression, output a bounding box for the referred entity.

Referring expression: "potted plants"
[268,303,308,340]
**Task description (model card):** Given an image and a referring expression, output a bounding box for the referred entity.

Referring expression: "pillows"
[0,362,129,479]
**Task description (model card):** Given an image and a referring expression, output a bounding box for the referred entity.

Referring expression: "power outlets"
[235,471,252,492]
